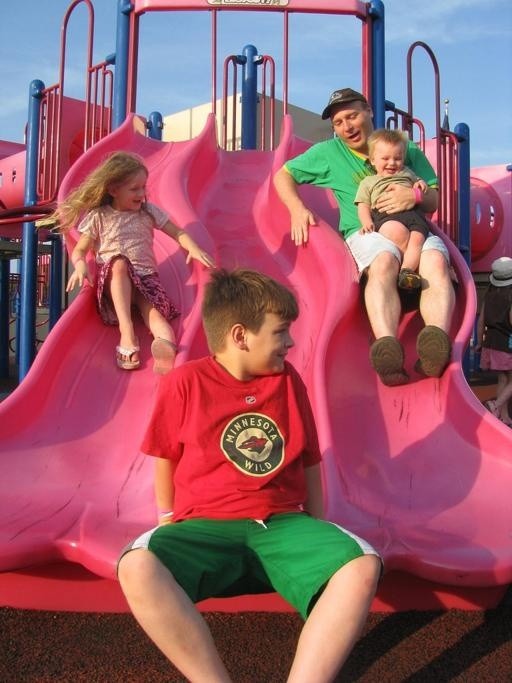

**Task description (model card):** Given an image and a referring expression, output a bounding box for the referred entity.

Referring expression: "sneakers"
[399,267,420,289]
[414,327,449,378]
[370,334,410,386]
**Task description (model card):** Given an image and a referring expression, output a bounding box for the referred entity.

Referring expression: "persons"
[115,269,385,683]
[53,152,219,376]
[353,128,429,306]
[474,256,512,428]
[274,87,457,387]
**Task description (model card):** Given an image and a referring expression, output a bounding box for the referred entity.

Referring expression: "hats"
[322,88,367,119]
[489,256,512,287]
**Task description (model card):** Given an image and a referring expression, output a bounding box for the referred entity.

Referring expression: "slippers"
[115,335,142,369]
[151,337,175,374]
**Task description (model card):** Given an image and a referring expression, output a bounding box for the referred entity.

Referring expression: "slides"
[0,109,511,591]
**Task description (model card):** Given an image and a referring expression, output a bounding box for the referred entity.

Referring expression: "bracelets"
[411,187,424,205]
[159,510,172,519]
[73,257,86,267]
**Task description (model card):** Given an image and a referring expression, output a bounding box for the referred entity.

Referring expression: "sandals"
[484,400,512,427]
